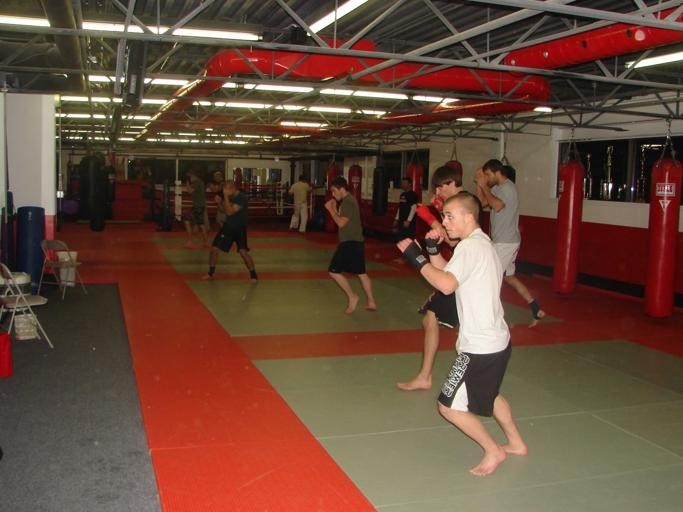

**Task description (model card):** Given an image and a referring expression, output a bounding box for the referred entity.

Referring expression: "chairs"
[0,237,89,351]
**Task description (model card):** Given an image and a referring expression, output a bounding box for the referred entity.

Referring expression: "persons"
[209,170,226,229]
[185,169,209,247]
[323,176,377,315]
[391,193,528,476]
[391,159,546,389]
[287,175,312,236]
[202,181,257,284]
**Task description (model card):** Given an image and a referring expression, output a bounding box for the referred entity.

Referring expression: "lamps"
[306,0,367,37]
[56,67,476,145]
[2,13,263,41]
[534,106,552,113]
[624,52,682,69]
